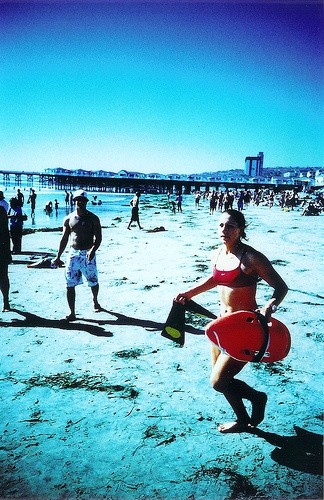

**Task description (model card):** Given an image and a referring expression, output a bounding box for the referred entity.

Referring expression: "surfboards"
[207,311,293,362]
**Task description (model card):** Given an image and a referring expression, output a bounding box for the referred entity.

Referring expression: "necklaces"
[235,242,241,247]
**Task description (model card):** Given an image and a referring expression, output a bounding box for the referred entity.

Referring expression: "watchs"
[268,303,277,312]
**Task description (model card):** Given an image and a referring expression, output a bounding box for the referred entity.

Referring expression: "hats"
[72,190,89,202]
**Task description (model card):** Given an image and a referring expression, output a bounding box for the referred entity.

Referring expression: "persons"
[51,190,102,319]
[70,192,73,206]
[0,191,9,212]
[17,189,24,207]
[11,198,23,253]
[177,209,289,432]
[44,199,59,211]
[64,190,70,207]
[0,204,12,312]
[127,192,143,230]
[27,190,37,213]
[194,186,324,216]
[175,193,183,213]
